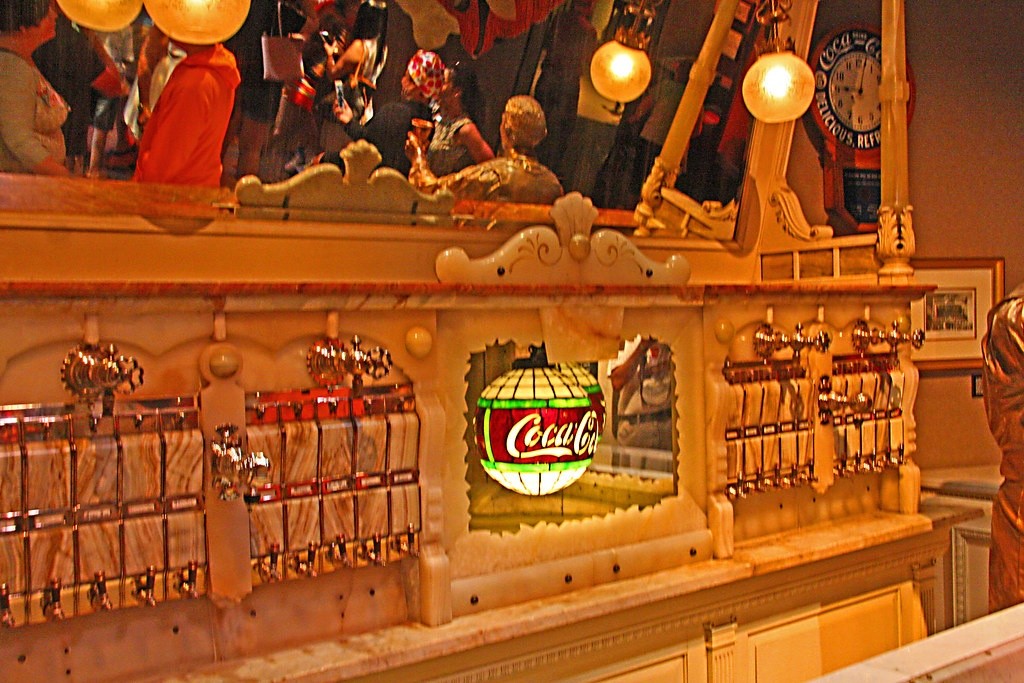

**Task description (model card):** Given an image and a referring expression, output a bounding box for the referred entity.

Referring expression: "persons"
[0,0,73,174]
[980,281,1024,614]
[405,96,563,206]
[32,0,704,206]
[607,334,673,482]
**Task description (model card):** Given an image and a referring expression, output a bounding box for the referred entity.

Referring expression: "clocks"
[806,21,916,151]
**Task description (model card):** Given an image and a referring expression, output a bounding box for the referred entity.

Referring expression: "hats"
[407,49,444,98]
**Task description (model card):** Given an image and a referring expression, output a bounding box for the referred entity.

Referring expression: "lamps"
[590,0,652,104]
[741,0,814,122]
[55,0,252,45]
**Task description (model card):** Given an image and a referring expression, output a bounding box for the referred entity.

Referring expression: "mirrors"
[1,0,818,229]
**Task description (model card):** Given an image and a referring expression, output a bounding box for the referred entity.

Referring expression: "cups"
[413,118,434,144]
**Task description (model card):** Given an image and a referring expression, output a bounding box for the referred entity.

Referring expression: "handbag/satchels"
[261,0,304,82]
[320,40,364,125]
[291,74,319,112]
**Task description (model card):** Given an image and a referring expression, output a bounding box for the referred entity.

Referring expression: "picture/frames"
[907,257,1006,370]
